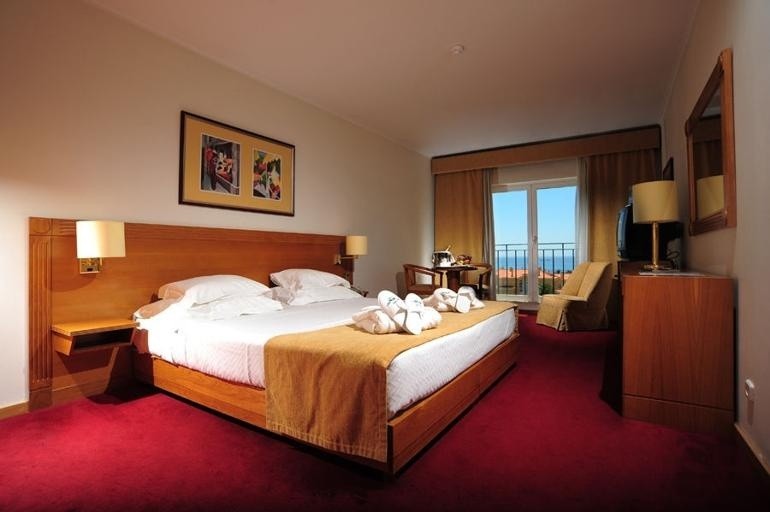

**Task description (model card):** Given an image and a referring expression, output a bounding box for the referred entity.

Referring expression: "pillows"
[189,295,283,322]
[157,274,272,309]
[270,268,351,292]
[264,286,363,306]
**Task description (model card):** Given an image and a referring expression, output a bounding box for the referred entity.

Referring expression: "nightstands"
[52,319,140,356]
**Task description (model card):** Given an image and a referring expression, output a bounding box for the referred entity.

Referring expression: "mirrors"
[685,47,737,232]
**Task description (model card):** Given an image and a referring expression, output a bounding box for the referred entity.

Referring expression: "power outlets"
[744,378,755,401]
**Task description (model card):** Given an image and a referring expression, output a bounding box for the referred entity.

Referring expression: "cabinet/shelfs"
[618,259,674,274]
[616,268,735,435]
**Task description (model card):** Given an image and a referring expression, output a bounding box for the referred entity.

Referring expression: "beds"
[26,216,521,476]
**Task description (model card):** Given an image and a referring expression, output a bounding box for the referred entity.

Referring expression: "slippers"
[404,292,425,335]
[455,286,476,313]
[377,289,411,335]
[434,286,458,313]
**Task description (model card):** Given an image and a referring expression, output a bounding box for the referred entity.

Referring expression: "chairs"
[459,263,493,300]
[553,261,614,331]
[403,264,444,297]
[535,261,592,330]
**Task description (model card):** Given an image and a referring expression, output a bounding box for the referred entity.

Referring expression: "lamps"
[76,220,126,274]
[696,175,725,222]
[334,235,368,265]
[631,181,681,269]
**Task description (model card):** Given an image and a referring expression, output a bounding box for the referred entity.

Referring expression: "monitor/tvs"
[616,205,684,259]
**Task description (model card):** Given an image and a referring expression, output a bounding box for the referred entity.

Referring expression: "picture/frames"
[179,109,295,218]
[662,157,674,181]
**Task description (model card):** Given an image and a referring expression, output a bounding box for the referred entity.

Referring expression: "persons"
[209,152,219,190]
